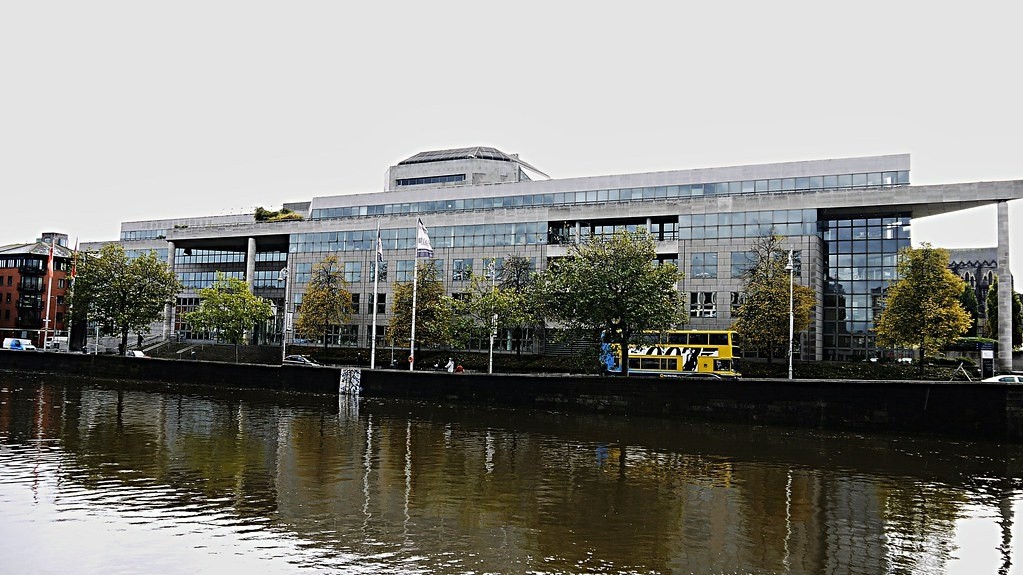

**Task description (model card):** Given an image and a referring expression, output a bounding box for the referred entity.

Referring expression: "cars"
[3,332,69,353]
[282,353,326,367]
[925,335,994,366]
[978,371,1023,382]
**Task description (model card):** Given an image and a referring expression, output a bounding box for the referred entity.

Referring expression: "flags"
[46,240,54,278]
[376,228,384,263]
[414,218,434,259]
[70,239,78,279]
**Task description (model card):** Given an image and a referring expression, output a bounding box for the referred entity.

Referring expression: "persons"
[455,362,464,374]
[136,331,144,348]
[445,357,455,374]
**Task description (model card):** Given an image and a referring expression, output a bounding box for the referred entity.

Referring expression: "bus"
[599,325,740,380]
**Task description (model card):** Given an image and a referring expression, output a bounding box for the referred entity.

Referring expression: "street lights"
[785,247,796,379]
[279,268,289,361]
[486,255,497,373]
